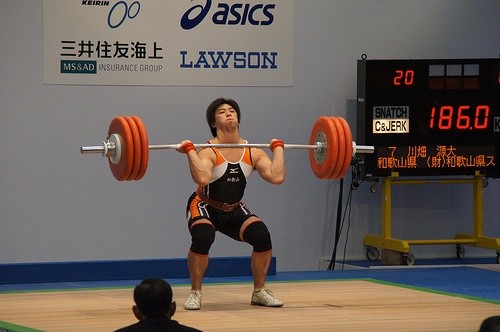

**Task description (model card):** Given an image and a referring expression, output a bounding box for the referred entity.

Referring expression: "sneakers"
[184,289,202,310]
[251,289,284,307]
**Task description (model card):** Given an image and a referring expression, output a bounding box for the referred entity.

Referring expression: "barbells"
[81,116,374,180]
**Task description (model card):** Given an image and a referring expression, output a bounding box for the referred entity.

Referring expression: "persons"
[112,279,203,332]
[178,98,285,310]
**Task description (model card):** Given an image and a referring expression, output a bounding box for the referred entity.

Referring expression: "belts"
[196,186,243,212]
